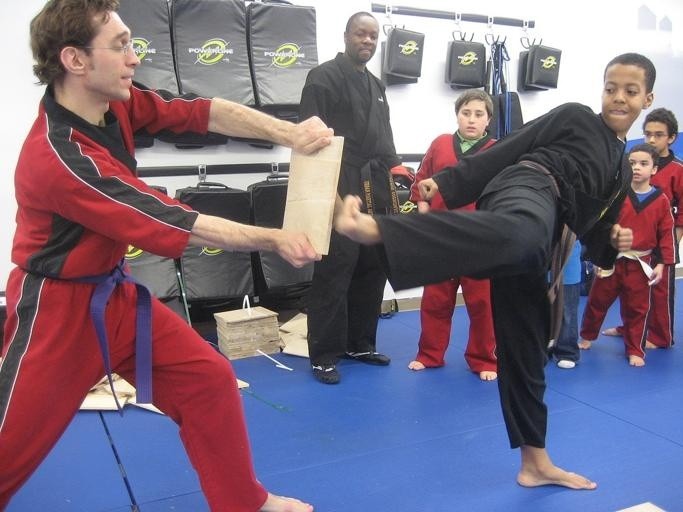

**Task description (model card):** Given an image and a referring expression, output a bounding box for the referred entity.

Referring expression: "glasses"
[68,38,135,56]
[642,131,672,139]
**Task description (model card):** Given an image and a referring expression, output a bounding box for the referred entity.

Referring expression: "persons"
[295,10,415,386]
[544,232,582,371]
[0,0,316,512]
[602,107,682,349]
[576,142,679,367]
[333,51,656,490]
[407,88,501,382]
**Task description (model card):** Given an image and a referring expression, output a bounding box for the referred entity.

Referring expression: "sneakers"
[340,347,390,367]
[309,360,341,386]
